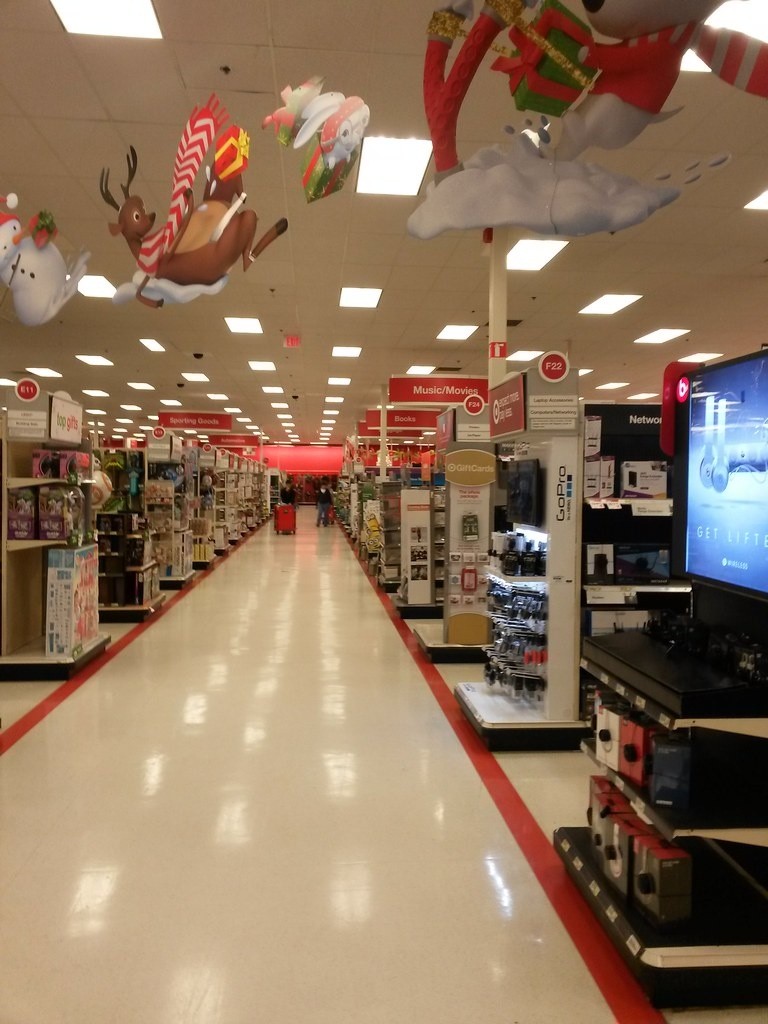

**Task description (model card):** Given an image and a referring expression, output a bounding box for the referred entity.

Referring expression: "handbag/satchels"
[326,505,336,521]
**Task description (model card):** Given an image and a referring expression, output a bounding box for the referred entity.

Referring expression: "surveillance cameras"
[292,395,299,399]
[177,383,184,388]
[193,353,203,359]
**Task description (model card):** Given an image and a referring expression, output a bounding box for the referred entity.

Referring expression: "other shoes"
[324,523,327,527]
[316,524,319,527]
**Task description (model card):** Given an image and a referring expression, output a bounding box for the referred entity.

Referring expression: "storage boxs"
[583,542,672,636]
[194,542,215,561]
[583,416,614,499]
[8,486,88,542]
[229,524,241,538]
[97,511,160,606]
[33,449,90,479]
[160,531,194,577]
[620,460,667,500]
[215,527,229,548]
[45,543,100,658]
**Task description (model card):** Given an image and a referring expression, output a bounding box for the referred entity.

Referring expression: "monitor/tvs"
[505,458,541,526]
[669,348,768,613]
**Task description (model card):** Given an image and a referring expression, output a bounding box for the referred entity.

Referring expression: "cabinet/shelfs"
[146,459,196,591]
[380,481,446,621]
[95,448,167,624]
[581,496,694,597]
[190,532,218,571]
[207,468,281,556]
[0,437,111,682]
[554,630,768,1011]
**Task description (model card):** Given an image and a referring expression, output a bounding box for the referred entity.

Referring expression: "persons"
[316,482,333,527]
[280,480,296,535]
